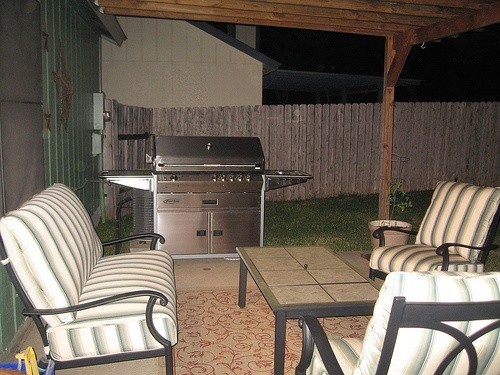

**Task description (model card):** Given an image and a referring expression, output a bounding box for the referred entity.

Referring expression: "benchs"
[0,183,178,375]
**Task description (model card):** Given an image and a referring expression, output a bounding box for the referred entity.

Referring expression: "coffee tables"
[236,244,380,375]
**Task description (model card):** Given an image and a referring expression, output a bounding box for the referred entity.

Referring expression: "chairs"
[369,181,500,282]
[294,267,500,375]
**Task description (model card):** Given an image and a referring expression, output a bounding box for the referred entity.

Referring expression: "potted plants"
[370,181,414,249]
